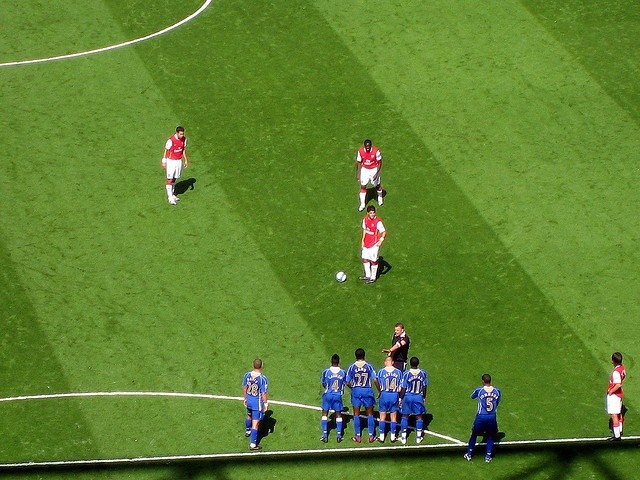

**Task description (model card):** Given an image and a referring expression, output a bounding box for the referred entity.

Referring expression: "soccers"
[335,272,346,282]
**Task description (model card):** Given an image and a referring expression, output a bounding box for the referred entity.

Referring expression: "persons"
[379,357,402,443]
[607,351,626,442]
[402,358,428,445]
[464,373,501,463]
[321,354,347,443]
[359,206,387,284]
[161,127,189,205]
[382,323,410,371]
[355,139,384,210]
[243,358,268,450]
[348,349,381,443]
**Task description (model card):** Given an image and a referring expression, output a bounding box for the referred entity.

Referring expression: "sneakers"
[485,457,493,463]
[391,436,396,443]
[365,278,375,284]
[319,437,328,443]
[378,197,383,206]
[398,437,407,444]
[464,453,472,461]
[607,437,621,441]
[173,194,180,201]
[351,435,362,443]
[376,436,384,444]
[169,198,177,205]
[358,276,370,281]
[358,203,366,212]
[416,436,424,444]
[368,435,377,443]
[250,445,262,450]
[245,433,250,437]
[337,437,341,443]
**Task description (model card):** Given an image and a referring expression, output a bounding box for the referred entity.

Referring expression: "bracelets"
[380,236,384,242]
